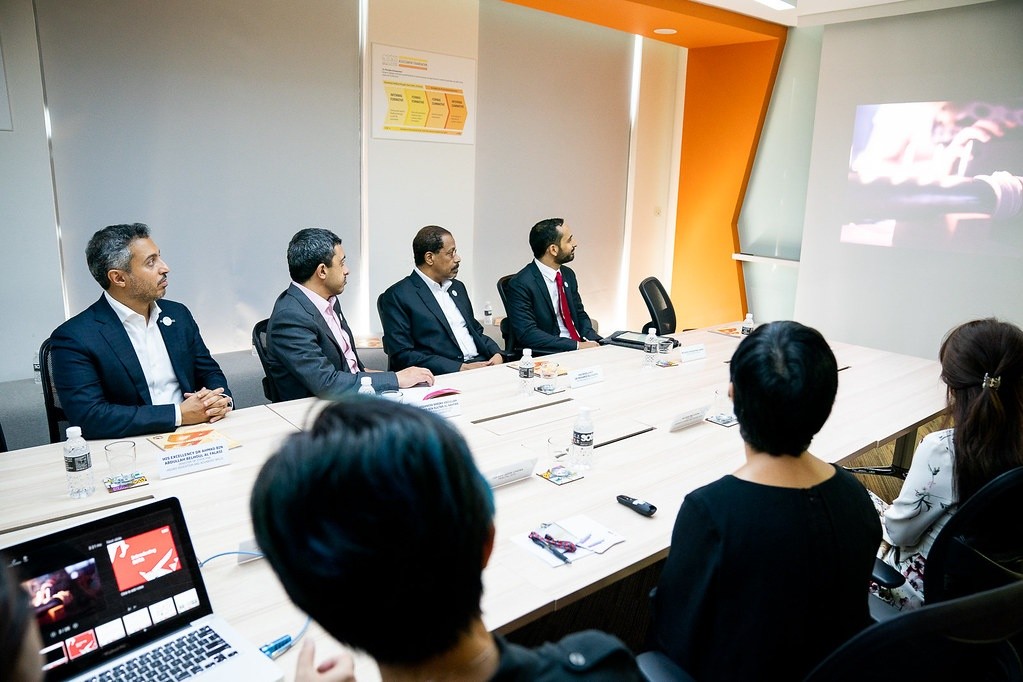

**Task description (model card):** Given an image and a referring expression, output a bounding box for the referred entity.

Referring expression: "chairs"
[38,336,73,445]
[496,274,563,363]
[804,466,1023,682]
[252,318,280,405]
[639,276,696,336]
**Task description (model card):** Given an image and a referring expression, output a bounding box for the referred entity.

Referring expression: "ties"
[554,272,581,343]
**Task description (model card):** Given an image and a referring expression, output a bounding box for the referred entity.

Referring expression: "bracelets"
[220,395,232,407]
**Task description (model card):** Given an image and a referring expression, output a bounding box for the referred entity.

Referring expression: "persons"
[868,318,1023,610]
[266,228,434,400]
[644,321,882,682]
[31,565,103,619]
[505,218,603,351]
[377,225,504,375]
[51,223,236,440]
[249,394,644,681]
[892,102,1023,244]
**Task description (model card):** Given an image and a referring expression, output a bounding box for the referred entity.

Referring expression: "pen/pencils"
[531,536,572,564]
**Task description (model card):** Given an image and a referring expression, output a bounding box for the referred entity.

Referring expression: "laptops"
[0,496,285,682]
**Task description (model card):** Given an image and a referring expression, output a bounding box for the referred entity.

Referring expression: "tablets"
[611,330,674,346]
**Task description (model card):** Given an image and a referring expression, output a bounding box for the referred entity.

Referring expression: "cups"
[548,435,573,476]
[540,361,560,392]
[658,342,673,362]
[382,390,403,404]
[105,441,136,483]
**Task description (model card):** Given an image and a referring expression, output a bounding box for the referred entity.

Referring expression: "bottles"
[358,377,376,394]
[742,314,754,339]
[64,426,93,499]
[520,349,534,395]
[573,410,593,472]
[645,328,657,367]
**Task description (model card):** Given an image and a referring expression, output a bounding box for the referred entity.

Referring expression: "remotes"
[617,495,657,516]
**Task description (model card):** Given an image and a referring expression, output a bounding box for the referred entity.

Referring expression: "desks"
[0,322,958,682]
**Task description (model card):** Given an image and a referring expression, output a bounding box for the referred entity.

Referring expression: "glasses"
[451,248,457,256]
